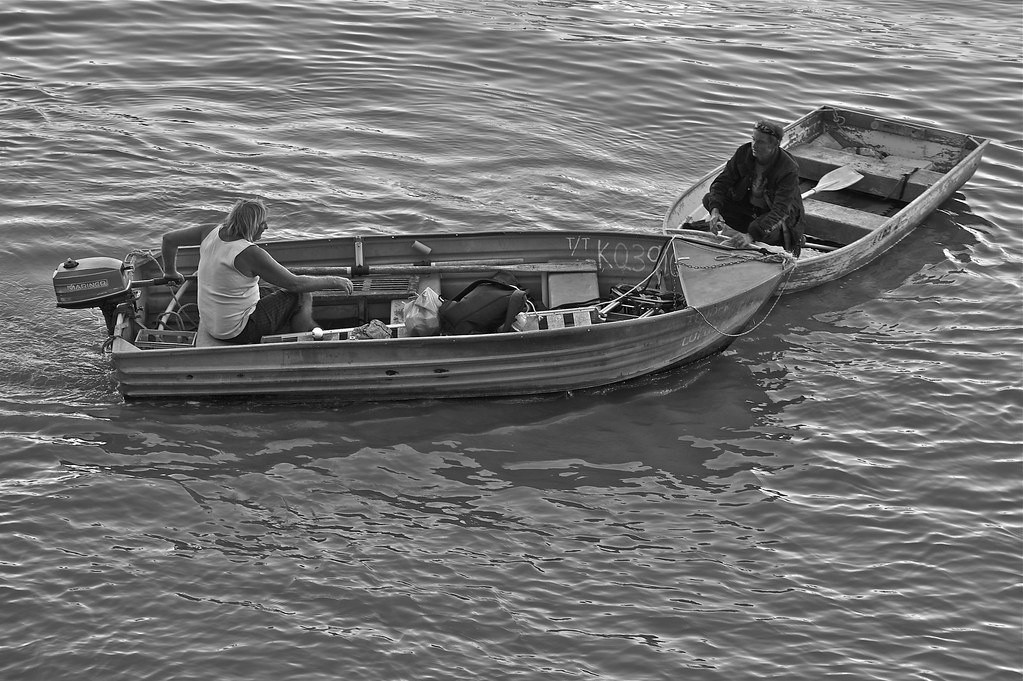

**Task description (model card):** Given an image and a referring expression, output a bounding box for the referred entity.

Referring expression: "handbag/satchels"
[403,299,440,337]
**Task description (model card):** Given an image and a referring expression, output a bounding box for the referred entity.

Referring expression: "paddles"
[281,257,598,276]
[798,163,864,200]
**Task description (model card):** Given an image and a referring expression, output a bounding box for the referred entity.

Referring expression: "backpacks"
[435,270,528,335]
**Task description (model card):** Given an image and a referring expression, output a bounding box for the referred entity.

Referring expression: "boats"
[52,229,801,399]
[661,103,991,298]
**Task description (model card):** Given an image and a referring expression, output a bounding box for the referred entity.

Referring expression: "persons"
[161,201,355,346]
[702,122,808,257]
